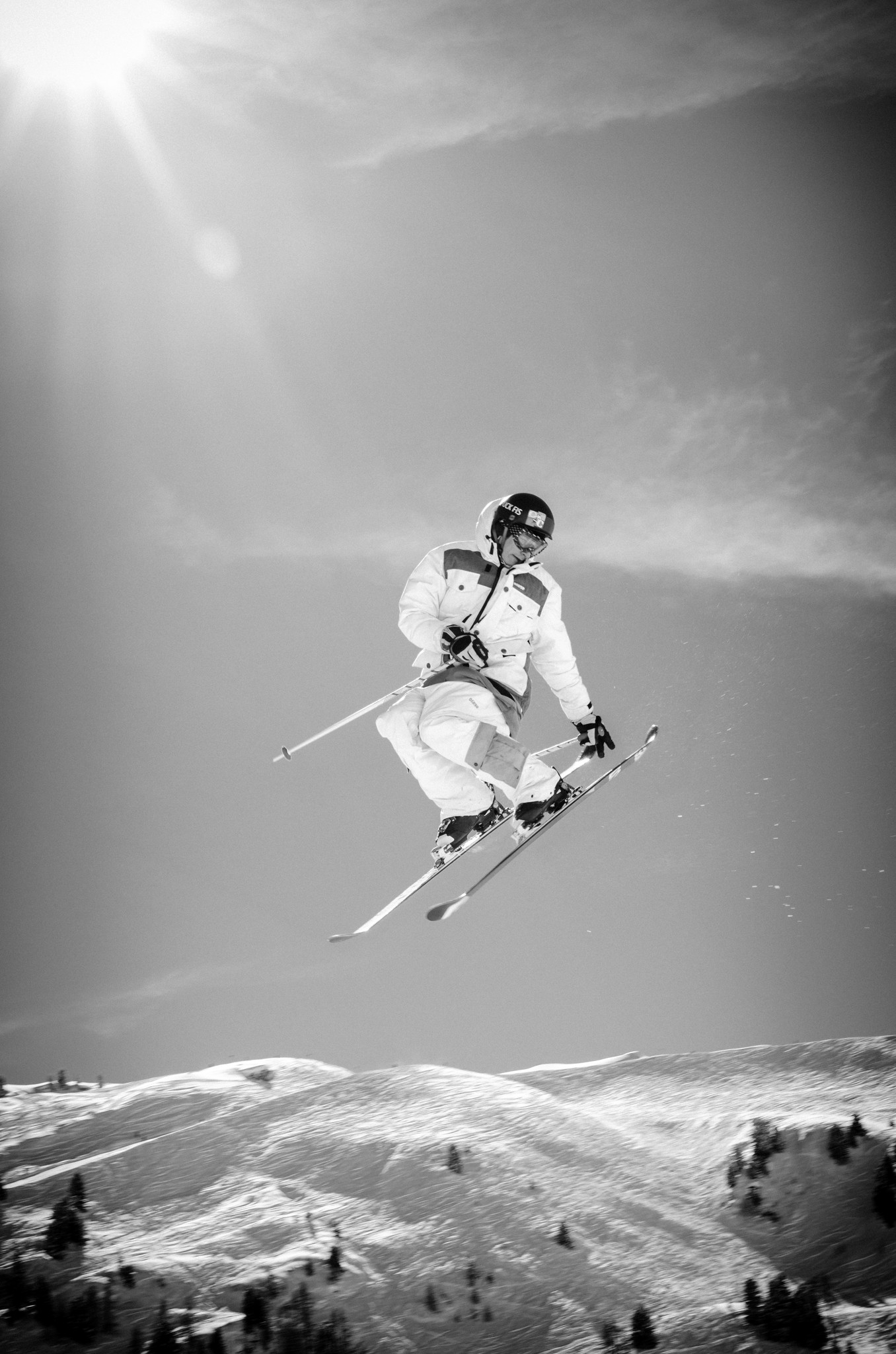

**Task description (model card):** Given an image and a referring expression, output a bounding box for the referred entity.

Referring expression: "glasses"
[513,528,548,557]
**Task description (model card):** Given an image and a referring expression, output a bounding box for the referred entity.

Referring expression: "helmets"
[496,493,555,541]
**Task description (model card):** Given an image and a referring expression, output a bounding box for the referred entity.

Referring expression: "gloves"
[441,624,488,669]
[571,714,615,758]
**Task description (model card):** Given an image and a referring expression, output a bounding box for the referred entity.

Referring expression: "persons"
[375,492,617,866]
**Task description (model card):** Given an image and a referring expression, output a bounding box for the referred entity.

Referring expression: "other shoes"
[515,779,571,822]
[434,800,499,847]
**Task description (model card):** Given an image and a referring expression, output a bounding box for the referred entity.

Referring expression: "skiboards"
[326,722,659,942]
